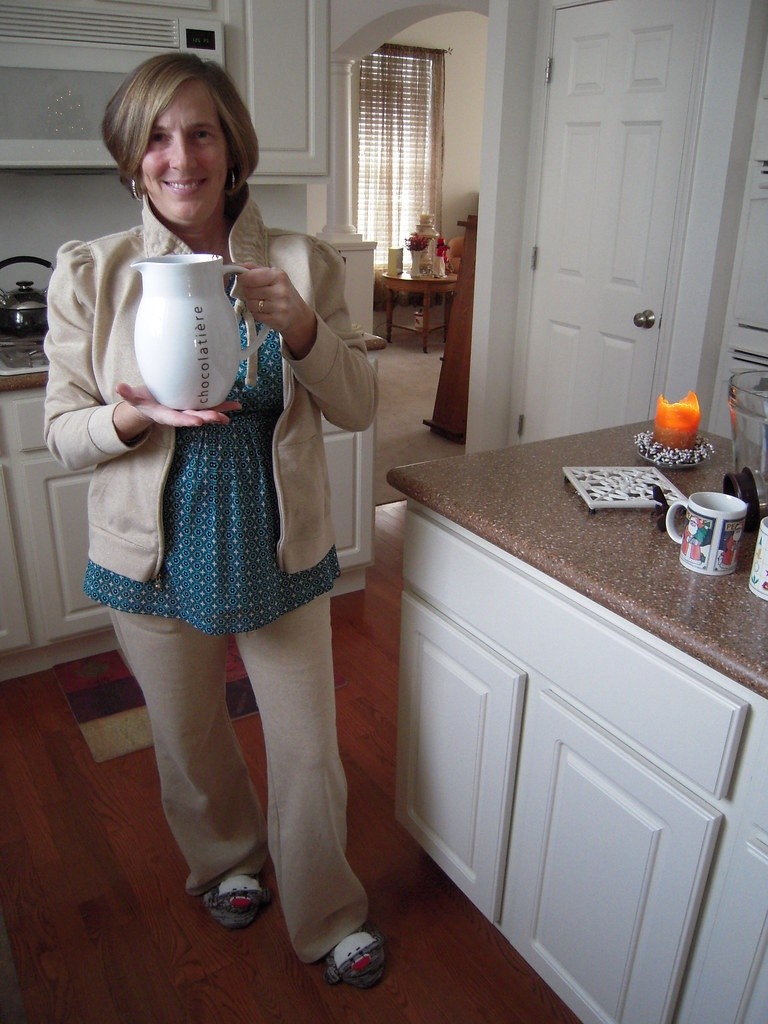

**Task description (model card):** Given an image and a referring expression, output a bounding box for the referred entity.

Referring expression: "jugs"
[130,254,269,409]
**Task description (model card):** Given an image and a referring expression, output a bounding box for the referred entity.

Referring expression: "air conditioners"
[0,0,226,77]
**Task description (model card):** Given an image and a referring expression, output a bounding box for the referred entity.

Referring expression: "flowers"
[404,233,434,251]
[436,238,450,258]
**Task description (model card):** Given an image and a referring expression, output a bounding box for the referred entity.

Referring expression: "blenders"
[723,370,768,528]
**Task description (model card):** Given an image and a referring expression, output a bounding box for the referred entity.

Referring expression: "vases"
[432,258,446,277]
[411,251,428,276]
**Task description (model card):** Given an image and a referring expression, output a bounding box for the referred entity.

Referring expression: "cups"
[747,516,767,600]
[665,491,748,578]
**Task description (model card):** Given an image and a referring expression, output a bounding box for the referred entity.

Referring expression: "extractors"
[1,0,227,176]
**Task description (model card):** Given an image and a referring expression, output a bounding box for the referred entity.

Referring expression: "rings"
[258,300,264,313]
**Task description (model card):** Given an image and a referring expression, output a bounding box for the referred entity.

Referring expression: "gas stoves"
[0,333,50,376]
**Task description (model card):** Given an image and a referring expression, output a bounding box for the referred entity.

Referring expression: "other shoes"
[324,919,393,987]
[202,869,270,927]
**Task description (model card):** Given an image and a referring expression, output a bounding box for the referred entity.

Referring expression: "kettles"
[0,256,54,336]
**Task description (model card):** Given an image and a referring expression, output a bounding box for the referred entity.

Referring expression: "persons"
[42,53,396,993]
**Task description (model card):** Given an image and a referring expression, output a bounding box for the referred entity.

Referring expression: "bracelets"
[116,431,146,444]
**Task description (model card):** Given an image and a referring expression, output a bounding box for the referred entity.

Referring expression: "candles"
[654,389,703,448]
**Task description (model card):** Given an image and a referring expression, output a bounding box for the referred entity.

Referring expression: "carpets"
[53,631,345,759]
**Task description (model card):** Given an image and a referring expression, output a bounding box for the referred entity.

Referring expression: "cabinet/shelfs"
[0,355,377,660]
[397,499,768,1024]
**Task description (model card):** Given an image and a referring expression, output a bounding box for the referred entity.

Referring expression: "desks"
[382,271,459,353]
